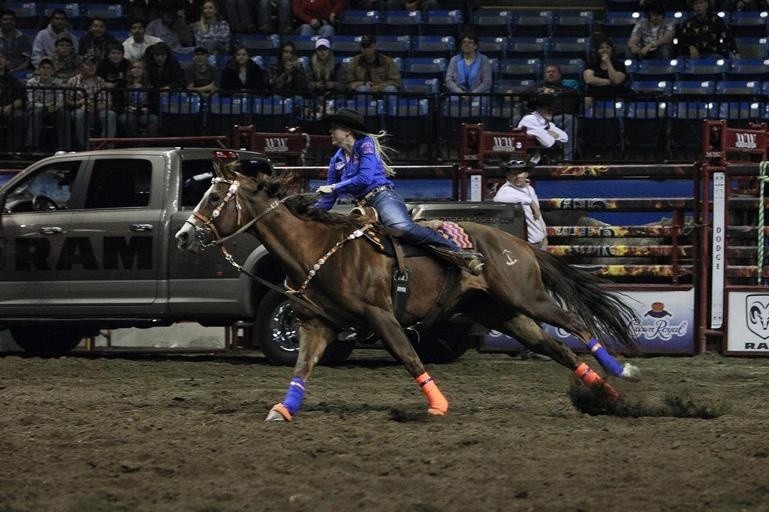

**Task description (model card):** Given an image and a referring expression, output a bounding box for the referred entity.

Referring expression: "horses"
[174,157,646,423]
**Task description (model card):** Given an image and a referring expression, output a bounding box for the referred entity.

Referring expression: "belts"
[355,185,389,207]
[530,239,544,245]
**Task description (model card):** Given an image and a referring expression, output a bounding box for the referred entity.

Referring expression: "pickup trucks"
[0,146,528,366]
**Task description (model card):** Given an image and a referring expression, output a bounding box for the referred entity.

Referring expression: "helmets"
[314,37,331,49]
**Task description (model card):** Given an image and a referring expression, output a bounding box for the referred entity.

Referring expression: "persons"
[673,0,743,59]
[303,38,346,112]
[308,109,484,276]
[580,37,629,107]
[511,63,584,163]
[264,39,307,114]
[445,34,492,115]
[514,92,566,165]
[292,0,345,39]
[629,1,674,61]
[346,30,400,115]
[1,1,265,156]
[495,158,555,363]
[256,0,292,36]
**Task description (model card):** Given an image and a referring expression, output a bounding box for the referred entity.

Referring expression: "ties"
[543,120,550,130]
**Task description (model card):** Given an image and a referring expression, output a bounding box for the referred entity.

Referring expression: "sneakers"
[461,250,482,274]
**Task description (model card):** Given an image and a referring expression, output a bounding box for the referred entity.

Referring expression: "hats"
[79,54,96,66]
[358,34,376,48]
[526,93,564,116]
[500,154,536,170]
[193,44,208,53]
[321,107,370,133]
